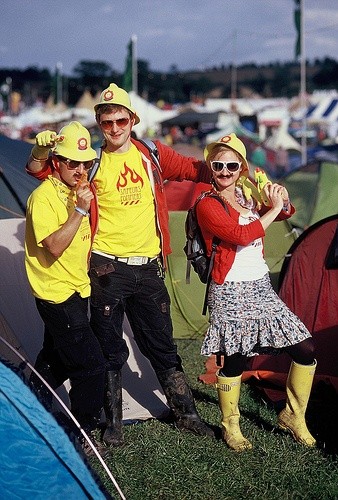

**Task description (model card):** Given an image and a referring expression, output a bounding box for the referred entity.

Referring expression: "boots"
[155,367,220,438]
[215,368,253,450]
[278,360,317,447]
[100,370,124,445]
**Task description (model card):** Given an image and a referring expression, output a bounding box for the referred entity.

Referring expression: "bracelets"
[75,207,86,215]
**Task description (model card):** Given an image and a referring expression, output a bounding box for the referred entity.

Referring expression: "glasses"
[210,161,242,174]
[58,157,94,170]
[98,117,131,129]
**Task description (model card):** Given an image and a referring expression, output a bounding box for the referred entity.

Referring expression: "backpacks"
[183,190,231,316]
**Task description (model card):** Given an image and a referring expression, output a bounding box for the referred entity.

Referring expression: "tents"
[162,159,338,401]
[0,360,106,500]
[0,166,170,425]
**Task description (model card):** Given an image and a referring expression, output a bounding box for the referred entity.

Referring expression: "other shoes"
[80,433,107,457]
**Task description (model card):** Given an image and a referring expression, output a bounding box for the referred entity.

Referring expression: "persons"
[195,133,318,452]
[24,122,109,456]
[24,83,216,448]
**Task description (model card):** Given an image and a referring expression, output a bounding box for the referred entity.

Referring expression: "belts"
[92,250,158,266]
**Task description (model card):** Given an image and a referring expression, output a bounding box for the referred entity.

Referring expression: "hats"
[203,132,249,171]
[50,121,97,162]
[93,83,140,125]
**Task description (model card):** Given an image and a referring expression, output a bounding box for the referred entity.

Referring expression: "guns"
[253,166,290,207]
[52,135,65,144]
[76,171,89,190]
